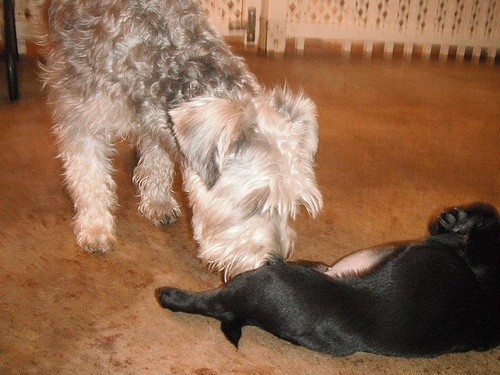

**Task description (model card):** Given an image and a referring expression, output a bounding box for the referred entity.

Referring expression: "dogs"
[158,203,500,358]
[27,0,325,281]
